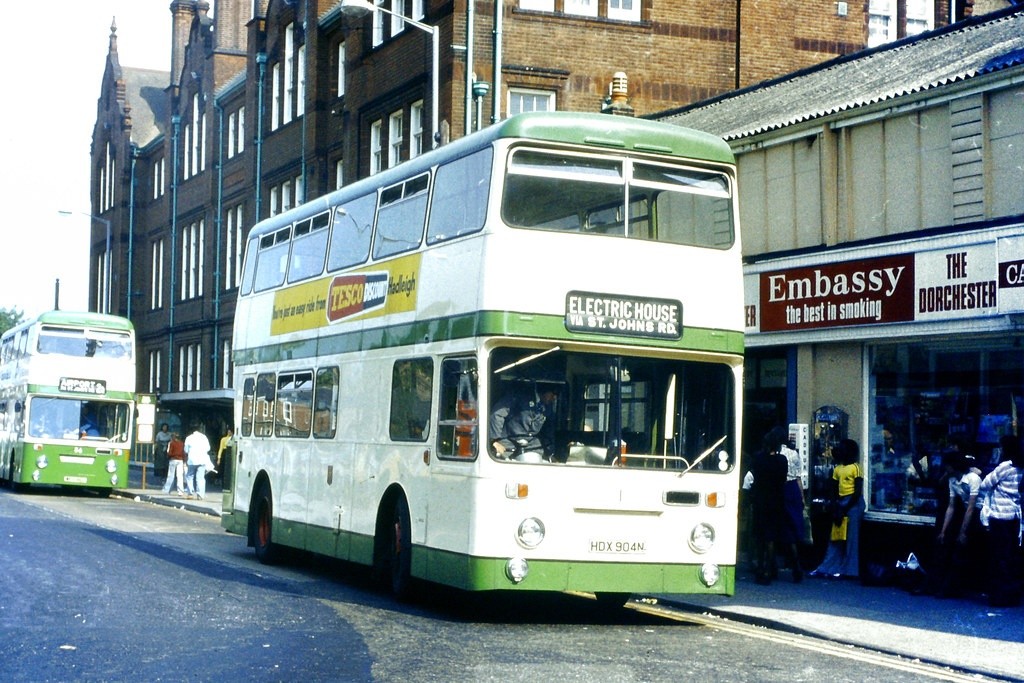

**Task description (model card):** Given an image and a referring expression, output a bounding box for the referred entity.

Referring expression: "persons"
[808,438,866,581]
[154,422,173,477]
[160,432,186,496]
[183,418,211,500]
[488,371,571,462]
[937,449,984,597]
[77,403,100,438]
[742,424,809,581]
[216,423,234,493]
[980,432,1024,607]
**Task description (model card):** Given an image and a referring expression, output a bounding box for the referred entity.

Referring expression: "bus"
[219,110,746,607]
[0,309,139,498]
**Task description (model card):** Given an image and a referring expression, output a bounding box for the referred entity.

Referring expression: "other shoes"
[196,494,205,500]
[748,562,769,585]
[807,570,829,579]
[186,493,194,499]
[791,565,804,583]
[825,573,850,581]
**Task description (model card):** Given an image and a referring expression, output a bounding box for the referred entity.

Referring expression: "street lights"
[339,0,441,151]
[59,209,111,314]
